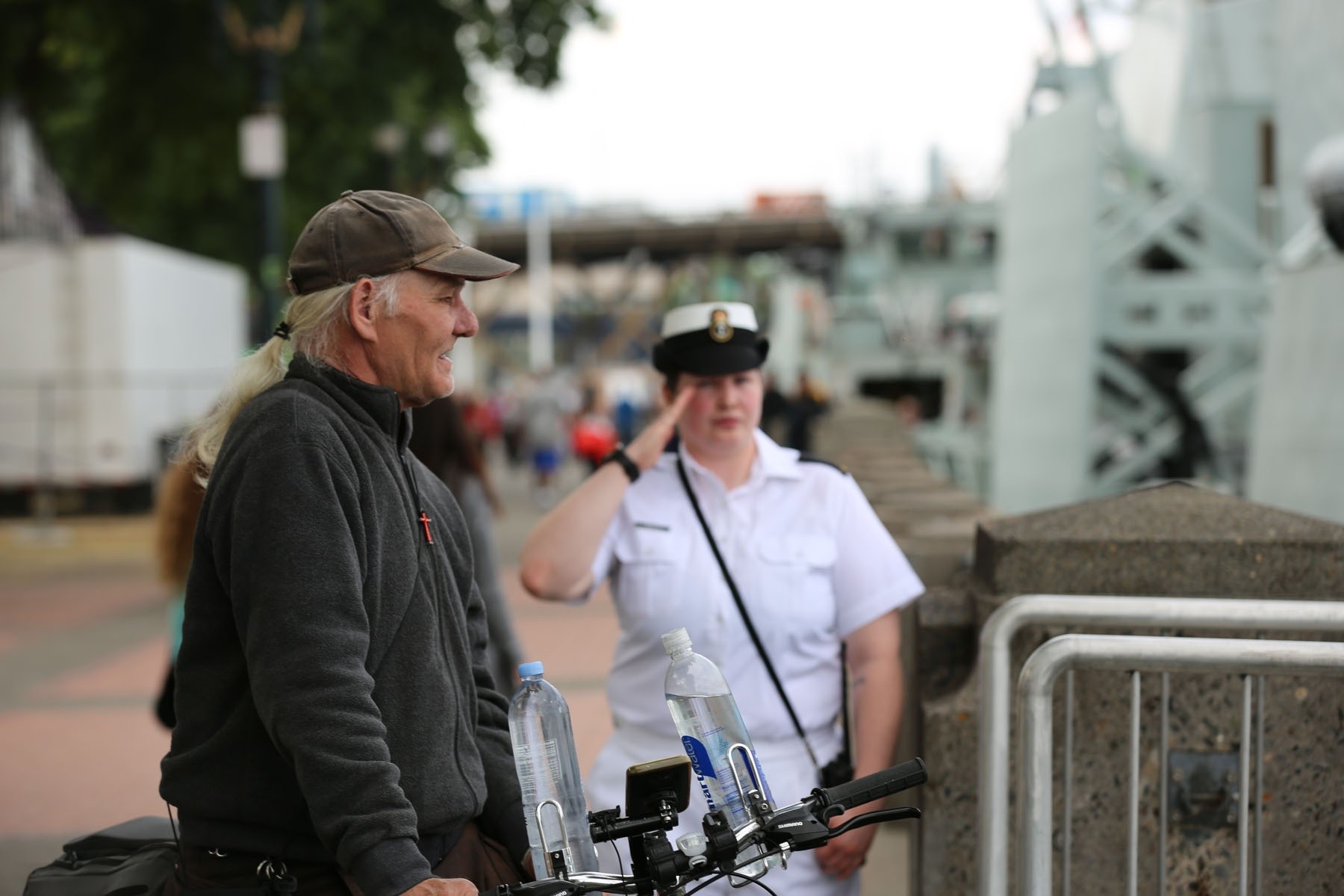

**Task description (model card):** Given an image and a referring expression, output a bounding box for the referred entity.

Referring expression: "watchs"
[600,439,641,483]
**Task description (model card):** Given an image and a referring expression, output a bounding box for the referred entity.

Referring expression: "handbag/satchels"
[817,751,853,785]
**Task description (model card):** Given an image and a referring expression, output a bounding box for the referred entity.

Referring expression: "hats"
[289,188,521,296]
[650,300,771,374]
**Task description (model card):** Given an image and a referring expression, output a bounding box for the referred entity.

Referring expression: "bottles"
[661,626,791,872]
[508,661,600,896]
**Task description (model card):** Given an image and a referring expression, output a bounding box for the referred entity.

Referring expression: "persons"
[517,295,926,896]
[435,346,842,523]
[150,188,553,896]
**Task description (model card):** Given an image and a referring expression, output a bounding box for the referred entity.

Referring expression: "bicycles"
[472,752,932,896]
